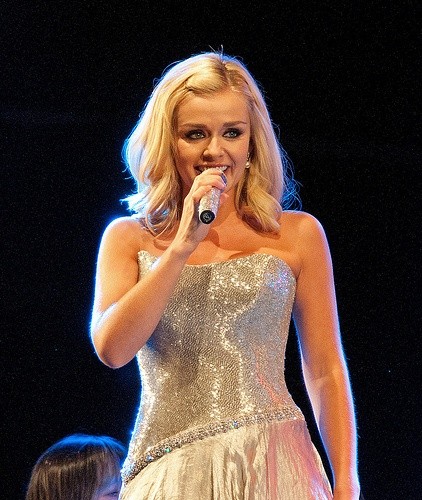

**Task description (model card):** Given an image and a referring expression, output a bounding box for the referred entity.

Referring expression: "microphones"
[199,168,228,225]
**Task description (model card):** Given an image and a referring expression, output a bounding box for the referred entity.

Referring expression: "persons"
[24,432,126,500]
[86,50,362,500]
